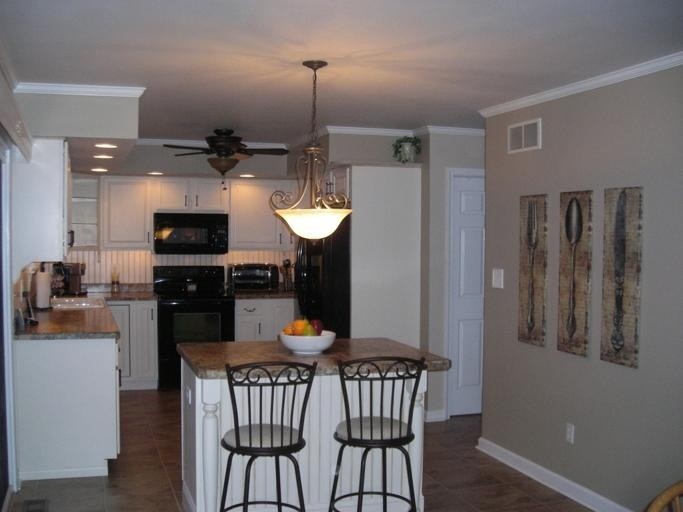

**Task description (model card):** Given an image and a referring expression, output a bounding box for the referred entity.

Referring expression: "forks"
[523,199,538,334]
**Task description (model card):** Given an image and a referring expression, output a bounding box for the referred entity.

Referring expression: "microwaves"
[152,211,228,255]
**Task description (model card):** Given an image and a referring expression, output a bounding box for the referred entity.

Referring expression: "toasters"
[227,262,279,291]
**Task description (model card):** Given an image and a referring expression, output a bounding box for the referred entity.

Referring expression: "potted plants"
[390,133,424,168]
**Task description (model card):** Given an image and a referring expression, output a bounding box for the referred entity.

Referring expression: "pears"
[303,325,317,336]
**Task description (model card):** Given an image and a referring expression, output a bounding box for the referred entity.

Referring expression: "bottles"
[281,259,292,293]
[111,266,121,294]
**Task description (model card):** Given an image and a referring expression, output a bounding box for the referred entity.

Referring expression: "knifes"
[611,189,628,353]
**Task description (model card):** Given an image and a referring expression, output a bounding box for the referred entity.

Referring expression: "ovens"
[158,299,235,393]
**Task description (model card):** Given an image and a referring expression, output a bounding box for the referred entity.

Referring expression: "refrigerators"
[291,208,350,343]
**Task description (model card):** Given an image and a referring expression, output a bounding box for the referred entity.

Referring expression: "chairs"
[324,353,429,512]
[216,358,320,511]
[643,478,682,511]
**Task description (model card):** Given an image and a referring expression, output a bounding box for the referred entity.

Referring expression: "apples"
[310,319,324,335]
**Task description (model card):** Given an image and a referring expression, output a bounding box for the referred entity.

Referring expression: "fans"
[158,127,290,162]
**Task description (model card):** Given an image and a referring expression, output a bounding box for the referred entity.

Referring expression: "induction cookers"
[153,284,235,300]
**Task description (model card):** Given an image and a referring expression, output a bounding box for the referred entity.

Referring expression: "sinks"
[50,296,104,311]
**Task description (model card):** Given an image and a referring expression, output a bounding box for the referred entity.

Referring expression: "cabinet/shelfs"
[232,295,298,344]
[227,174,313,252]
[9,135,71,265]
[316,164,351,202]
[68,169,99,249]
[149,175,228,215]
[105,298,160,393]
[96,175,152,253]
[10,334,121,482]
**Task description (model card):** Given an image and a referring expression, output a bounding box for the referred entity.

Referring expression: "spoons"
[565,196,582,340]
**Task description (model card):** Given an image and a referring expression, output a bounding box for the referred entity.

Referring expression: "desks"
[173,334,452,512]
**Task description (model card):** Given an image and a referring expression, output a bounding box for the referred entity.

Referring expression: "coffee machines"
[63,262,89,295]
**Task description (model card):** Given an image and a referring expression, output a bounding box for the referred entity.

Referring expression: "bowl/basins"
[279,331,337,354]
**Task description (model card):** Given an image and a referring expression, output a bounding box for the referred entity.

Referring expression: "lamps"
[205,154,241,177]
[270,59,352,243]
